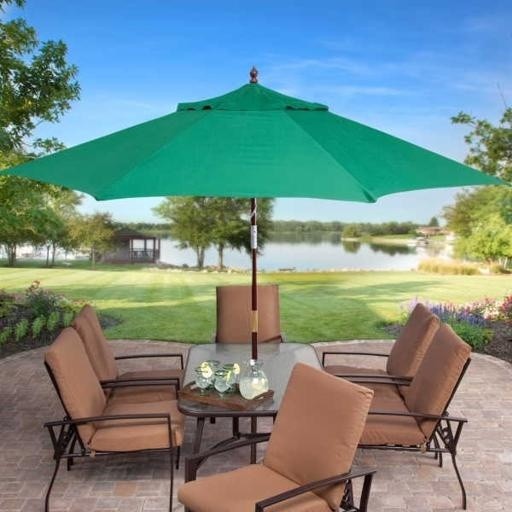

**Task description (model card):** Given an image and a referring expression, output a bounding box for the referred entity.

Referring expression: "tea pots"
[237,358,269,401]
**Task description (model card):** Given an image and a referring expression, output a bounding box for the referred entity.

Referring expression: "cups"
[194,359,239,402]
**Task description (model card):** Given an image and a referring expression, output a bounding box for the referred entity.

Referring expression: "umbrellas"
[0,65,507,465]
